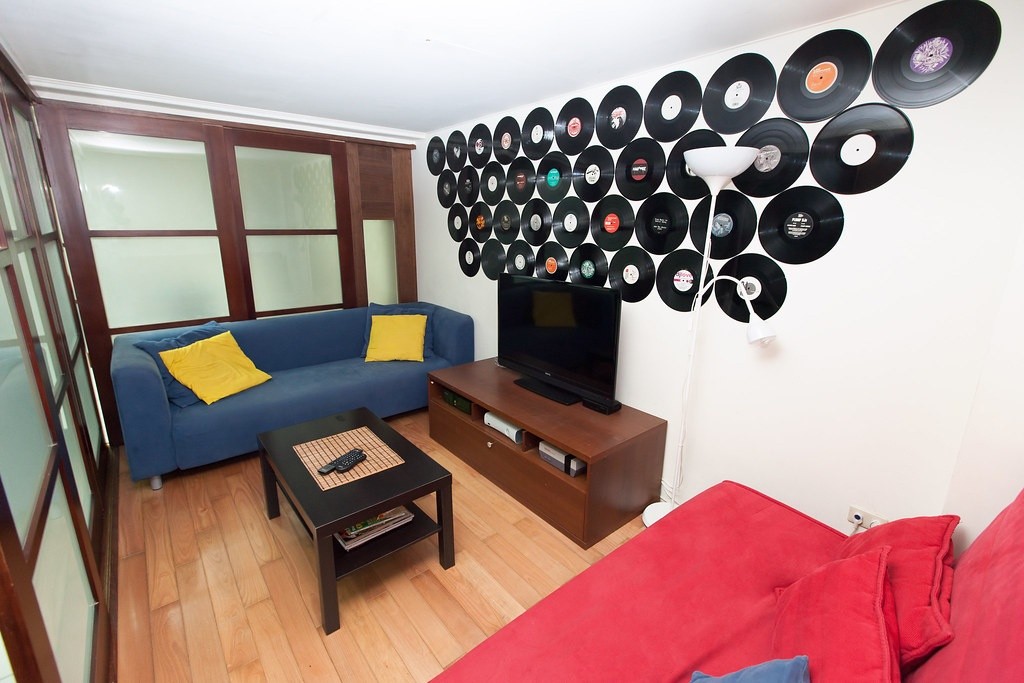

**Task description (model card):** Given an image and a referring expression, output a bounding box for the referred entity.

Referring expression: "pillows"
[689,654,810,683]
[361,302,438,359]
[134,319,225,409]
[834,512,961,666]
[365,313,429,362]
[159,330,274,408]
[771,541,900,683]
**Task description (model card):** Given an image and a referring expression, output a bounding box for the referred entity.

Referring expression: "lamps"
[641,143,781,527]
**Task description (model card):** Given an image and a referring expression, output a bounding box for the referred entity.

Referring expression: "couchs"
[432,481,1024,683]
[111,303,475,492]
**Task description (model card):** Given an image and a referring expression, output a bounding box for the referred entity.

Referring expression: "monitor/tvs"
[498,272,621,406]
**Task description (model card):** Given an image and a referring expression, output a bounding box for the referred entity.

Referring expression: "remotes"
[336,454,367,473]
[318,449,363,474]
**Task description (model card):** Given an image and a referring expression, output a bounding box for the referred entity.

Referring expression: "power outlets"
[845,505,889,530]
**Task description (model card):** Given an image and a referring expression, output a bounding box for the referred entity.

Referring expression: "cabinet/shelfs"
[427,358,670,550]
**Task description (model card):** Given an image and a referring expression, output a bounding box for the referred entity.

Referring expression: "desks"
[256,408,457,635]
